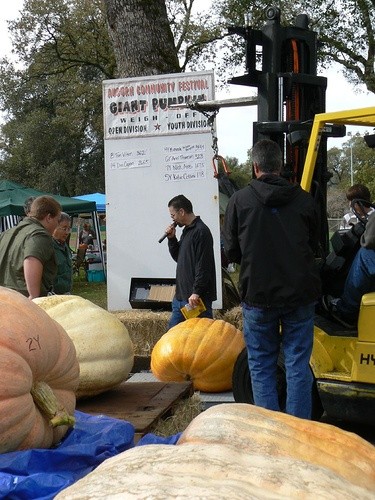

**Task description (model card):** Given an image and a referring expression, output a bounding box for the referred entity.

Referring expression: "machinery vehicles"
[167,6,374,416]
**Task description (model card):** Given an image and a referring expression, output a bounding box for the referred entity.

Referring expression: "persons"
[316,184,375,329]
[166,195,217,329]
[79,221,96,250]
[17,197,37,224]
[223,139,330,420]
[51,212,73,295]
[0,195,58,300]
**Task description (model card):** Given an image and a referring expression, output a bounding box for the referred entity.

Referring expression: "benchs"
[358,291,375,342]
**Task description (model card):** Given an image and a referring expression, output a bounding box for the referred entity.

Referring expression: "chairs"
[72,248,88,275]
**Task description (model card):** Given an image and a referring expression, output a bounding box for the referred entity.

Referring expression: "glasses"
[171,210,179,218]
[56,227,72,233]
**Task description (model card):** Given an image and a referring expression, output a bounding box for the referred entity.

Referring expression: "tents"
[0,178,107,285]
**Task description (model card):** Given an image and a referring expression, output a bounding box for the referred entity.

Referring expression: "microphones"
[158,222,177,243]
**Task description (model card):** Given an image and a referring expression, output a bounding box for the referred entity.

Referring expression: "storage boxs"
[87,270,104,282]
[88,261,104,271]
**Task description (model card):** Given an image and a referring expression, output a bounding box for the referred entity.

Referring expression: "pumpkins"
[150,317,246,391]
[49,444,375,500]
[30,295,134,398]
[0,286,80,454]
[176,402,375,497]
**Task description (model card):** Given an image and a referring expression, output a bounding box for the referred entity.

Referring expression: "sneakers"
[322,294,356,328]
